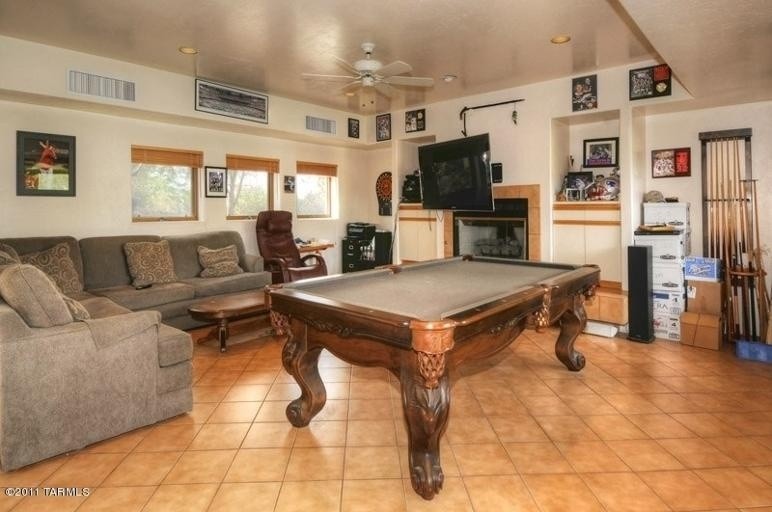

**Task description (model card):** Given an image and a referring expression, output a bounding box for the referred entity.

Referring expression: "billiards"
[535,307,548,327]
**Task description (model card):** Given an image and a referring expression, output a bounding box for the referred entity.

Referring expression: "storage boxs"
[634,202,724,351]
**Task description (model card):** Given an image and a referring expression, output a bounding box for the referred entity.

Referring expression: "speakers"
[375,231,392,267]
[626,246,656,344]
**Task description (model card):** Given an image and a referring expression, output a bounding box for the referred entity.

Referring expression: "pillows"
[122,240,178,288]
[195,243,246,278]
[1,240,89,330]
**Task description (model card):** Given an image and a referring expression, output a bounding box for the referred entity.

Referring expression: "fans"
[303,43,434,98]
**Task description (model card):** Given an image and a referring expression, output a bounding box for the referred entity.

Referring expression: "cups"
[564,187,580,203]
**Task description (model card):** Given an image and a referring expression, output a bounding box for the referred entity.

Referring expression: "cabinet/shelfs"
[553,200,620,336]
[398,200,437,265]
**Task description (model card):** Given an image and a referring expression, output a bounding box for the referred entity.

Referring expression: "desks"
[294,241,333,266]
[264,255,602,502]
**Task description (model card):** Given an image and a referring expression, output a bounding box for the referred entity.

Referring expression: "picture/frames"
[204,165,227,198]
[405,108,426,133]
[17,128,76,196]
[195,78,269,125]
[583,138,618,168]
[652,147,691,178]
[628,63,673,101]
[375,111,391,142]
[348,118,360,138]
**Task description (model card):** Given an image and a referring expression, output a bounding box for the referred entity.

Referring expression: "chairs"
[255,209,327,285]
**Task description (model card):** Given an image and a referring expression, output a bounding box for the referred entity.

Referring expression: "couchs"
[1,226,271,473]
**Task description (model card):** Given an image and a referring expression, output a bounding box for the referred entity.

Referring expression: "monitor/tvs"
[418,133,495,212]
